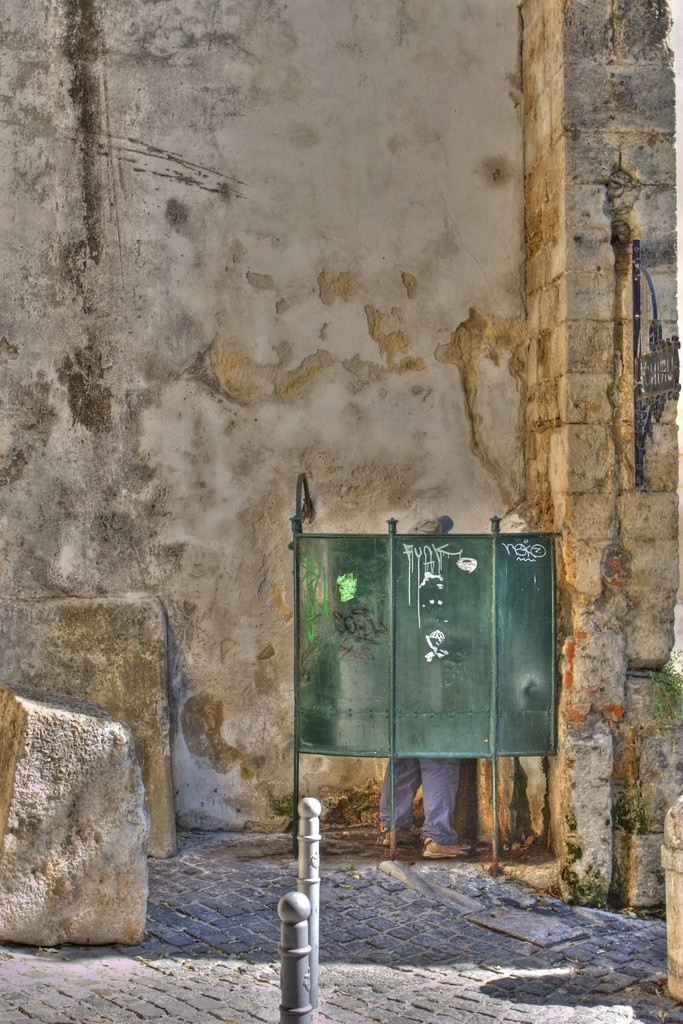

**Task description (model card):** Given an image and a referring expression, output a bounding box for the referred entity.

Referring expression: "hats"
[409,516,454,535]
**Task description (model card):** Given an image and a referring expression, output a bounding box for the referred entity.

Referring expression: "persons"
[377,515,476,858]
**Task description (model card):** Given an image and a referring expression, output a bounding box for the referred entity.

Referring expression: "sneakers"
[377,825,422,847]
[422,838,476,859]
